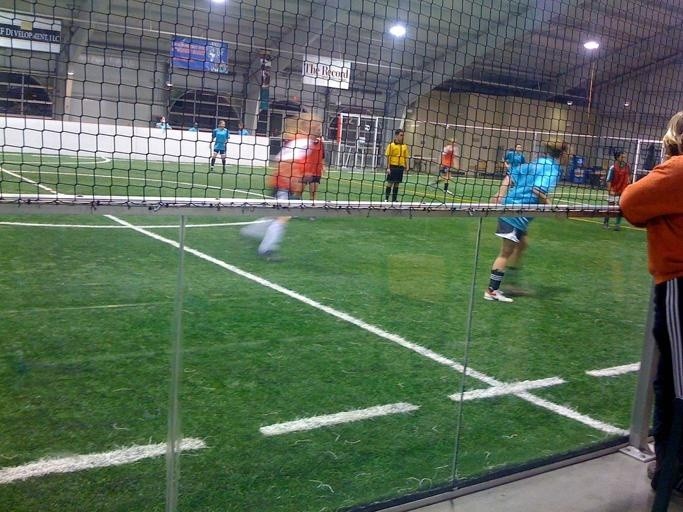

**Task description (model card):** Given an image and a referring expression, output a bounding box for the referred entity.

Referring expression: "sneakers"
[482,286,515,304]
[599,221,622,231]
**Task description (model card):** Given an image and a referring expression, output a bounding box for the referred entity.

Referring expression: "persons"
[384,129,411,203]
[504,144,526,173]
[155,114,171,129]
[601,150,632,232]
[188,121,200,132]
[236,121,249,135]
[483,138,574,303]
[438,137,456,195]
[209,120,230,174]
[618,111,682,499]
[267,113,326,200]
